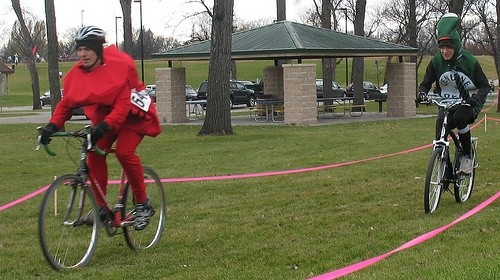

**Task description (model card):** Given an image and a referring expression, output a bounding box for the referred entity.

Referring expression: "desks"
[316,97,358,117]
[253,99,284,120]
[186,100,207,120]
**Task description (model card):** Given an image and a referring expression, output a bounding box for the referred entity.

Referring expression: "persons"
[416,13,490,190]
[40,26,163,231]
[14,53,18,64]
[36,53,39,60]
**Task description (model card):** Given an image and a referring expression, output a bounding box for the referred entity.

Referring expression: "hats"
[438,39,455,49]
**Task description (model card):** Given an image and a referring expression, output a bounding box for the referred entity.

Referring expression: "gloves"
[415,93,428,106]
[84,123,108,146]
[466,96,480,106]
[37,121,58,146]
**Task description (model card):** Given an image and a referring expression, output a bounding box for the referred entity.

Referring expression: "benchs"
[317,105,365,119]
[248,106,284,122]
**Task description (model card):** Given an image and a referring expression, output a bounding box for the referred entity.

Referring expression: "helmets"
[73,25,106,47]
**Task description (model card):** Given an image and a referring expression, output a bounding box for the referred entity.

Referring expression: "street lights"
[81,9,85,26]
[339,7,348,83]
[115,16,122,50]
[134,0,144,87]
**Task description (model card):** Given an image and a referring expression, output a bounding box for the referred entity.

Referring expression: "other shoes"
[133,204,157,232]
[83,212,93,225]
[459,152,476,174]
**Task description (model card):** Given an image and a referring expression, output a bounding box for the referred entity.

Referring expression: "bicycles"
[33,125,167,272]
[412,90,479,216]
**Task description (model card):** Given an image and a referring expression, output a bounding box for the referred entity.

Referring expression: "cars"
[185,85,198,101]
[39,89,65,106]
[198,80,256,111]
[316,78,347,102]
[144,84,156,104]
[346,82,387,100]
